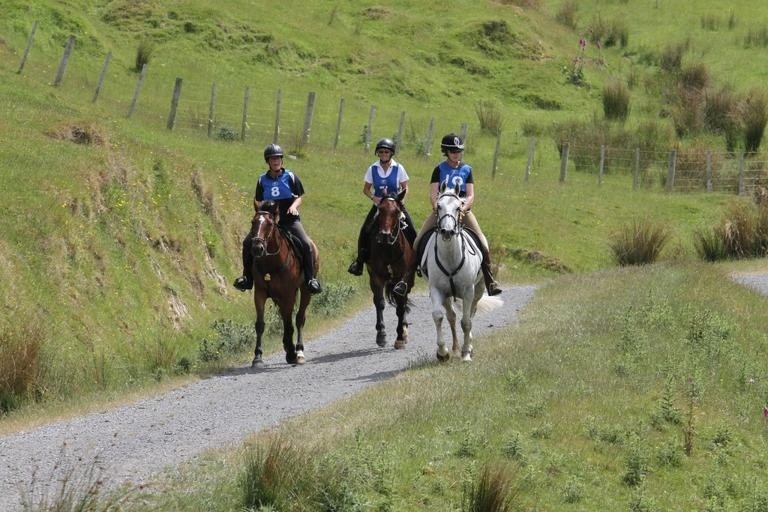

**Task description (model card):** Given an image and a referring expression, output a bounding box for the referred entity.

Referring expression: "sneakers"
[306,279,322,294]
[487,285,501,295]
[348,261,363,275]
[233,276,253,290]
[395,279,412,295]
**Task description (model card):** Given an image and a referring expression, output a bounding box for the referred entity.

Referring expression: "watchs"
[370,195,374,200]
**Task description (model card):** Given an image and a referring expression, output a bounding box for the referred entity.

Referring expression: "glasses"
[378,150,390,154]
[449,150,461,154]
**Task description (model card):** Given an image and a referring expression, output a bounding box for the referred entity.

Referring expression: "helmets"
[375,139,395,156]
[264,144,283,161]
[442,133,464,153]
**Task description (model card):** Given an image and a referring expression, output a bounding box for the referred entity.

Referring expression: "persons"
[393,132,503,298]
[348,137,428,277]
[233,144,322,293]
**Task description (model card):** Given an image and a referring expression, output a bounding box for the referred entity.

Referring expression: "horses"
[251,198,319,370]
[418,181,485,364]
[365,188,418,350]
